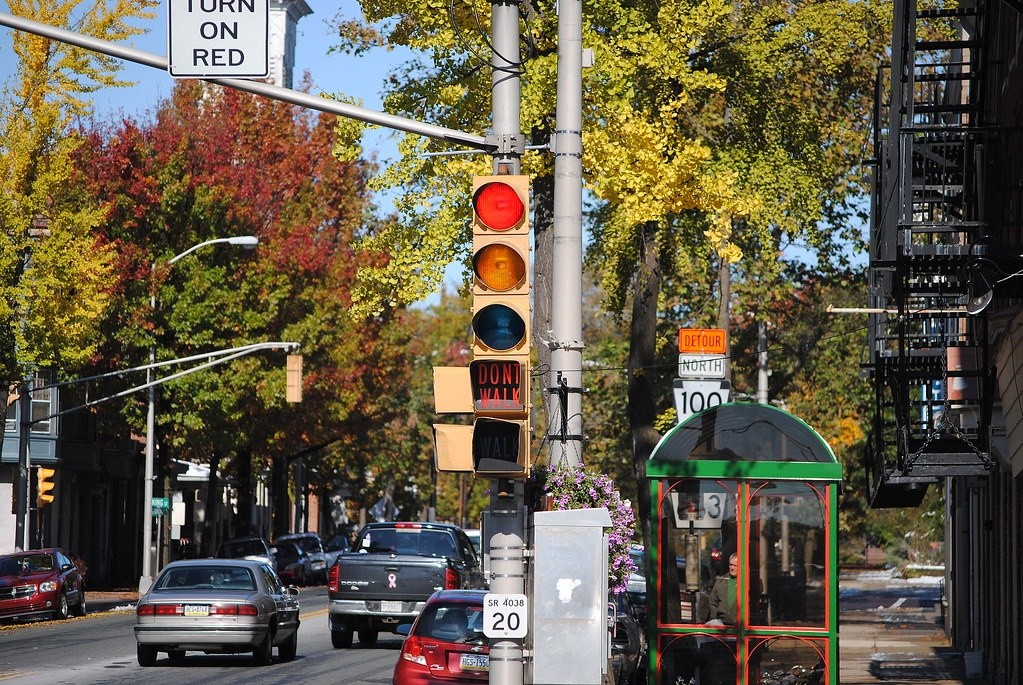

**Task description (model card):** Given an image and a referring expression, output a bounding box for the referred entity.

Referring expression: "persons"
[707,552,738,624]
[768,535,806,622]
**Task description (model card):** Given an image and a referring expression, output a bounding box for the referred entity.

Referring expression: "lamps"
[962,235,1023,315]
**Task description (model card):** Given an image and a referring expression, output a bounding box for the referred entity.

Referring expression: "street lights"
[140,232,259,603]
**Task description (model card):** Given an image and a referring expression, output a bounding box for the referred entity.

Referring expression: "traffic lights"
[472,174,530,481]
[35,468,56,508]
[6,379,22,407]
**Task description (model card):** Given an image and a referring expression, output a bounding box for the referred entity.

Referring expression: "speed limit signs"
[483,593,530,639]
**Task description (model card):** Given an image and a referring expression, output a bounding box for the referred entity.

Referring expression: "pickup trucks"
[326,521,488,649]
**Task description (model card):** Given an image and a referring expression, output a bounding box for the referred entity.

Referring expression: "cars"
[618,545,687,596]
[206,536,278,579]
[269,540,315,587]
[390,589,491,685]
[134,560,301,667]
[320,533,353,577]
[440,528,481,564]
[279,532,331,585]
[1,548,87,621]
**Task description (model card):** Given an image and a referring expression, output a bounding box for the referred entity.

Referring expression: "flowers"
[527,464,644,596]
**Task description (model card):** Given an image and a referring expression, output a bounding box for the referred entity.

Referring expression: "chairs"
[433,610,471,640]
